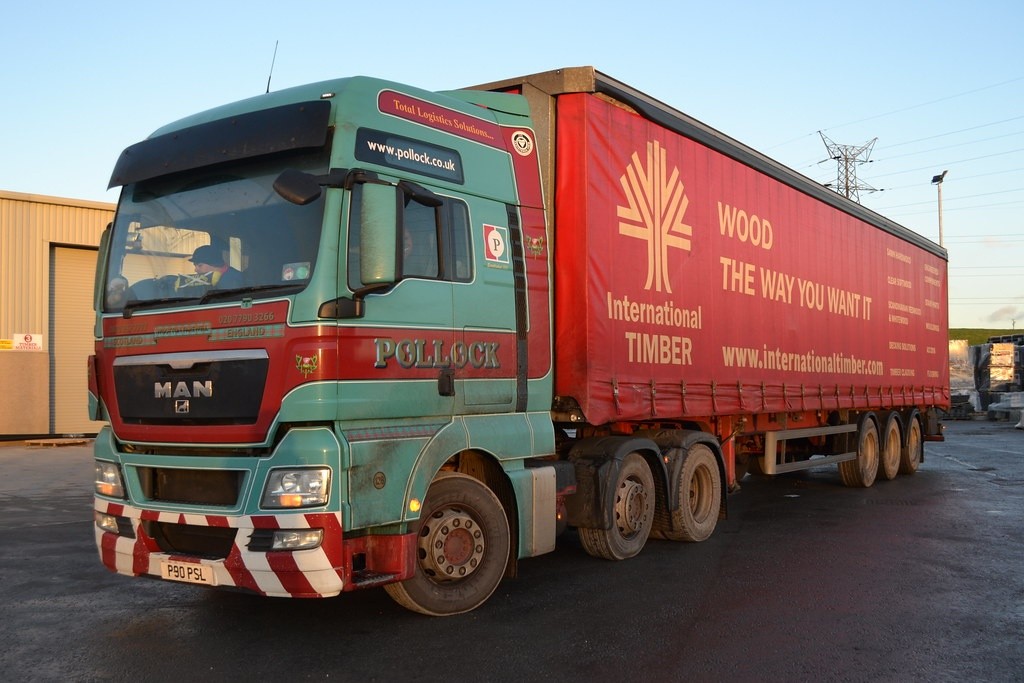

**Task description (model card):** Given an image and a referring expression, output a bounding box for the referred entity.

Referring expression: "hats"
[188,245,225,268]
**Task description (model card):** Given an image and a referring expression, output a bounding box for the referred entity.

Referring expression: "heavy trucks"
[82,62,953,619]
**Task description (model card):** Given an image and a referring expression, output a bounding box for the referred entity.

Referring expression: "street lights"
[932,171,948,246]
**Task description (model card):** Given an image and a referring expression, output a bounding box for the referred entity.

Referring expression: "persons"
[188,245,243,291]
[402,225,420,276]
